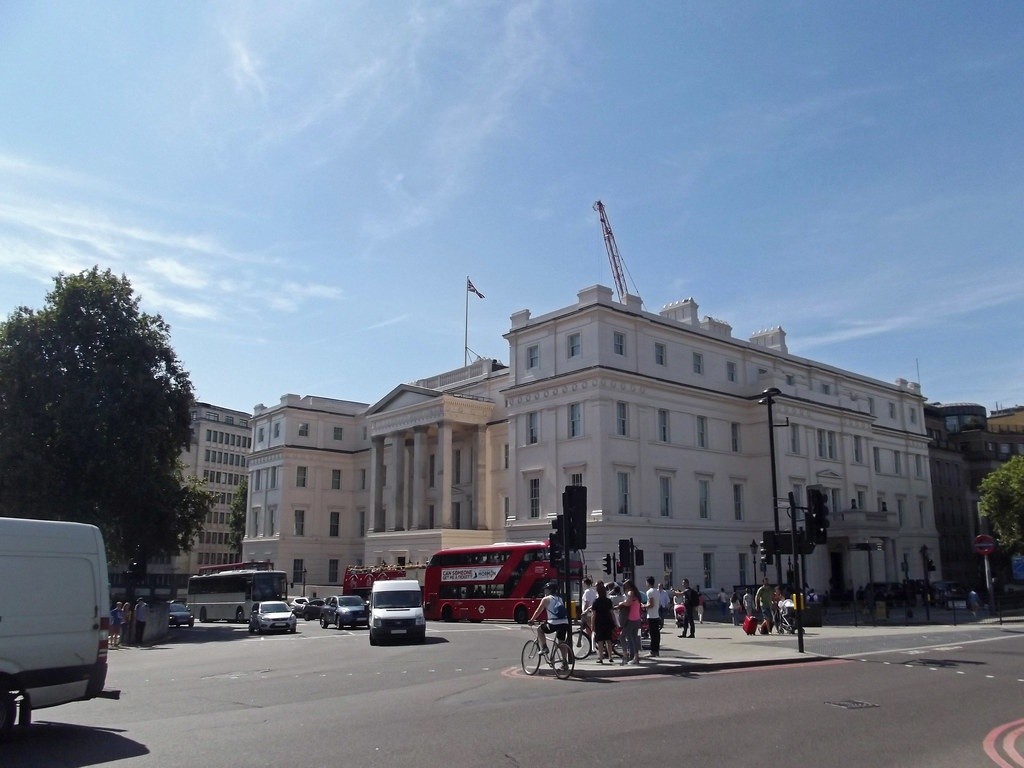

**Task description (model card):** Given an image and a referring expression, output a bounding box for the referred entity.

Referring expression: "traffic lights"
[760,540,773,565]
[602,554,611,575]
[810,488,830,544]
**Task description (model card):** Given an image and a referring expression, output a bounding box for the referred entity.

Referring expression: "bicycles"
[521,612,634,681]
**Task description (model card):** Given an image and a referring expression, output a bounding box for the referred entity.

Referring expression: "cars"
[301,598,325,621]
[319,595,368,630]
[170,602,194,628]
[291,597,313,609]
[249,602,296,636]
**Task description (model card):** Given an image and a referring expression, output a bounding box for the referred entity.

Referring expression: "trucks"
[369,580,427,647]
[1,518,121,741]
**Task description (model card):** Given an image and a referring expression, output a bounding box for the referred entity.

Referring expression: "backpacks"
[547,595,567,619]
[808,594,815,602]
[686,587,699,607]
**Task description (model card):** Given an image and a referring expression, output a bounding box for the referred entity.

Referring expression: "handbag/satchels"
[618,606,629,626]
[717,596,721,602]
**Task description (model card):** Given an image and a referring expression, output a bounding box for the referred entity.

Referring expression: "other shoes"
[687,633,695,638]
[596,660,603,664]
[559,664,569,669]
[538,648,549,655]
[621,659,627,664]
[609,659,614,663]
[644,651,656,657]
[677,631,687,638]
[628,660,639,665]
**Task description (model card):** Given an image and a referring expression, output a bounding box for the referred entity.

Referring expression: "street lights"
[750,539,759,585]
[302,568,307,597]
[758,386,784,593]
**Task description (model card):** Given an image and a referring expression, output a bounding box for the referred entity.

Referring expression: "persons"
[528,581,570,671]
[822,578,1000,620]
[348,554,540,599]
[109,599,150,647]
[579,575,816,666]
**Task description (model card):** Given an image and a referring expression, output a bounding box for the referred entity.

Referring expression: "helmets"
[543,582,557,589]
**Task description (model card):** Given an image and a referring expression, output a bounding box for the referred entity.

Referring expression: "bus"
[423,542,588,624]
[199,561,274,576]
[343,564,426,601]
[187,569,289,624]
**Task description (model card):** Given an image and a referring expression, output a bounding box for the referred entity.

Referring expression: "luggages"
[758,619,775,634]
[742,615,758,635]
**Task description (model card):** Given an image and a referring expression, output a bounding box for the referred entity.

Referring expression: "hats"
[592,580,604,588]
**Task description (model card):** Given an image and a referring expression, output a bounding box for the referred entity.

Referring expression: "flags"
[469,281,485,298]
